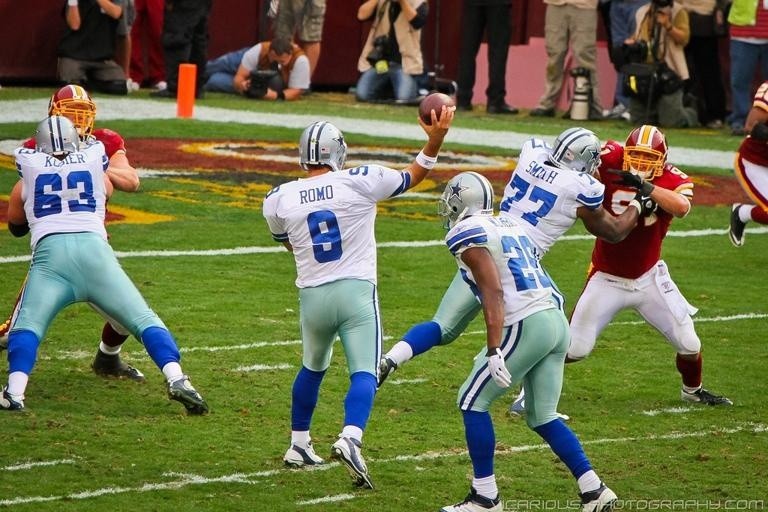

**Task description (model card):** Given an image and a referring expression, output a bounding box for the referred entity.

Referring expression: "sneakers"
[169,375,209,415]
[438,486,506,512]
[730,203,746,249]
[282,439,326,469]
[377,354,400,390]
[575,481,617,512]
[510,387,571,423]
[331,435,375,492]
[92,355,144,381]
[679,382,735,406]
[0,386,29,412]
[731,119,745,136]
[529,107,554,117]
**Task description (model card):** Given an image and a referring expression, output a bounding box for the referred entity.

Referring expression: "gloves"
[629,193,660,217]
[486,345,513,390]
[607,166,654,197]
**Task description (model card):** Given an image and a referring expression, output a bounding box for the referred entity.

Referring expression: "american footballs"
[418,92,456,125]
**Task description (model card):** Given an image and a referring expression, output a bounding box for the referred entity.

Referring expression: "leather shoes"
[151,85,206,101]
[455,103,473,111]
[486,103,520,115]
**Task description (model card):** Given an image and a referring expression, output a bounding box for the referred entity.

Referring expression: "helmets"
[437,171,496,224]
[298,121,347,171]
[620,124,670,182]
[551,126,601,175]
[31,115,79,157]
[47,83,98,139]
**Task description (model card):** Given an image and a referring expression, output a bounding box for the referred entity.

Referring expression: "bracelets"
[413,150,438,170]
[627,199,643,221]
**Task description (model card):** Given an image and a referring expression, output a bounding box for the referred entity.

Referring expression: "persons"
[262,104,454,492]
[728,79,768,247]
[378,127,659,389]
[0,114,207,418]
[566,124,736,409]
[436,169,618,512]
[0,85,146,384]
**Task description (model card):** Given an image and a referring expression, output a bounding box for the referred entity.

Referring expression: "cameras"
[366,36,387,67]
